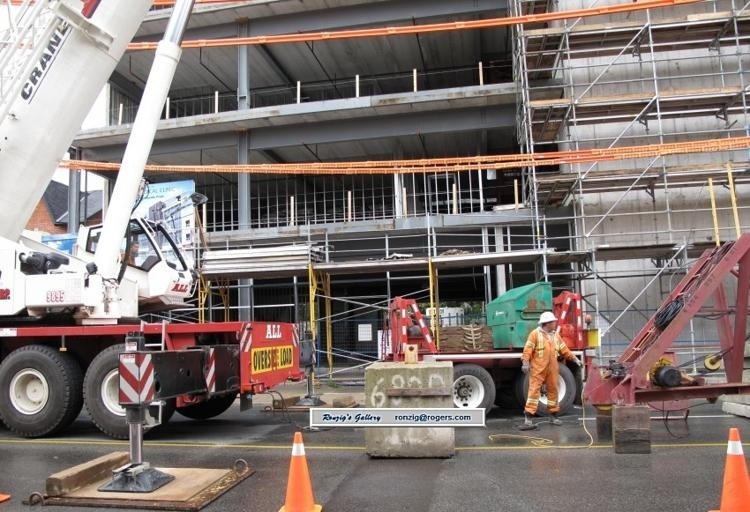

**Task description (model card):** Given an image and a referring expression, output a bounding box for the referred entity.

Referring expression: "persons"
[520,309,584,425]
[122,240,140,266]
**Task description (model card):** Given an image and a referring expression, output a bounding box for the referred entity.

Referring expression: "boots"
[523,411,535,427]
[548,413,562,426]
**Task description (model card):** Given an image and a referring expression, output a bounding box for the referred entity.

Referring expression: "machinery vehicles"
[0,0,326,494]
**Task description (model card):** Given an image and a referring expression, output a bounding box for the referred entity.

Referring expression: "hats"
[537,311,558,325]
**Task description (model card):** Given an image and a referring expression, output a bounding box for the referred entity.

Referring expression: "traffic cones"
[276,430,322,512]
[707,426,750,512]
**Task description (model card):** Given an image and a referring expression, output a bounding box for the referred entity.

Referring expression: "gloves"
[521,362,531,374]
[574,357,584,368]
